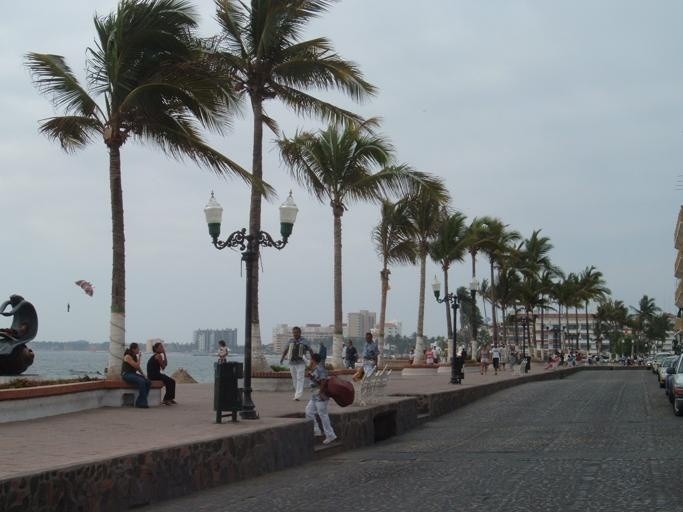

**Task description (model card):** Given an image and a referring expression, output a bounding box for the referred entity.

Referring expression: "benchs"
[252,368,358,391]
[0,379,163,424]
[438,362,481,375]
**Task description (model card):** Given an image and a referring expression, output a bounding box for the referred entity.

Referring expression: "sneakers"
[313,430,324,437]
[480,368,500,375]
[162,399,177,405]
[322,433,338,445]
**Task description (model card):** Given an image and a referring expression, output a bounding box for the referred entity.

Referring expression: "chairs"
[512,358,528,376]
[353,364,392,406]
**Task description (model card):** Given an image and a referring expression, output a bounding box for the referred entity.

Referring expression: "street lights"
[203,189,299,419]
[509,306,538,358]
[431,273,480,384]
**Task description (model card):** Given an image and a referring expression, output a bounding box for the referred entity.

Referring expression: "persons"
[279,327,313,400]
[119,342,152,407]
[479,344,518,375]
[318,341,326,369]
[304,353,337,445]
[216,339,228,364]
[344,337,358,369]
[407,350,415,365]
[423,346,440,364]
[363,356,377,377]
[147,343,178,406]
[360,331,379,372]
[542,349,599,370]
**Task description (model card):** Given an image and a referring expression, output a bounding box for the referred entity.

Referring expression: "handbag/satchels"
[352,368,365,383]
[308,374,355,407]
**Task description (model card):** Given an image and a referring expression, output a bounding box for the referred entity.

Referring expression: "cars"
[645,351,683,415]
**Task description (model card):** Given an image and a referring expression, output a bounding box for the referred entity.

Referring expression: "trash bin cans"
[524,357,531,374]
[213,360,244,424]
[451,356,464,385]
